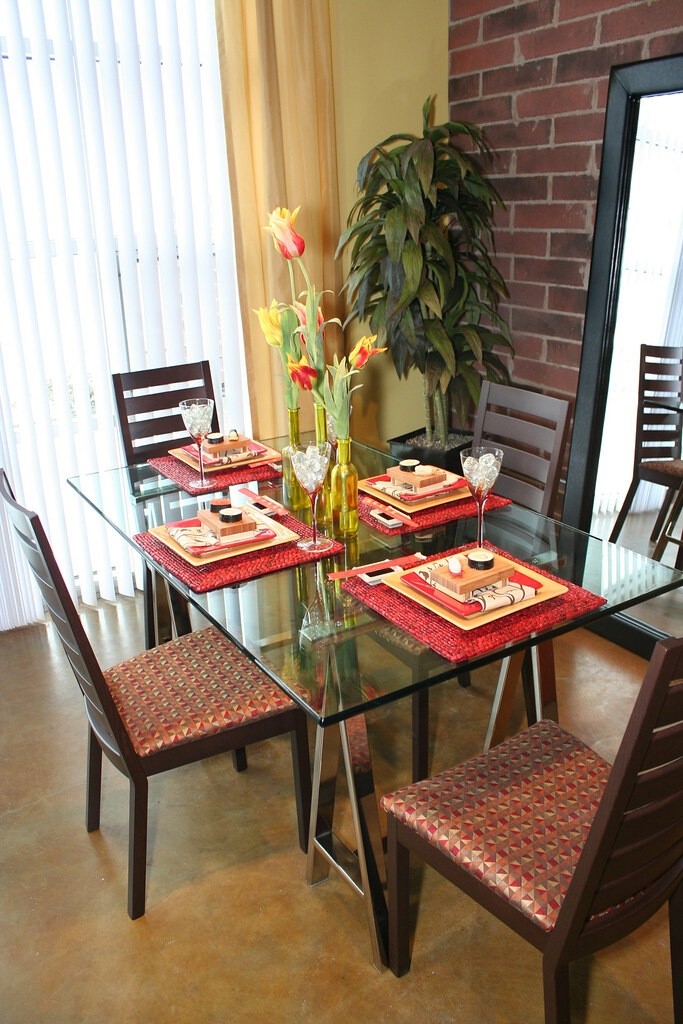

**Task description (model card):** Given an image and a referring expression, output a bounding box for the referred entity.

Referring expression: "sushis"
[207,432,224,444]
[467,551,494,570]
[210,498,242,523]
[448,557,462,574]
[415,465,435,476]
[399,459,420,472]
[228,429,239,440]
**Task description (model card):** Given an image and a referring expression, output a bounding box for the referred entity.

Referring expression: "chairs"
[1,468,314,921]
[605,344,683,547]
[112,358,233,646]
[343,378,569,786]
[379,636,683,1024]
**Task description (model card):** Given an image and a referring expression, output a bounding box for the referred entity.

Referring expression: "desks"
[68,419,682,980]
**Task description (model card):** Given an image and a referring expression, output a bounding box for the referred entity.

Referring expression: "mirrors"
[558,59,683,669]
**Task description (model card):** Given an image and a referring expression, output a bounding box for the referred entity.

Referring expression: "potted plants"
[339,94,519,549]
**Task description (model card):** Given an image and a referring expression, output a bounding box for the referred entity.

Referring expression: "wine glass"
[299,558,338,655]
[179,397,217,489]
[460,446,504,551]
[289,440,334,553]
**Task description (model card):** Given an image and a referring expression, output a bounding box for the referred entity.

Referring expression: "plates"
[164,516,276,555]
[365,476,468,502]
[167,438,282,473]
[179,440,267,464]
[147,505,301,566]
[400,572,543,616]
[358,465,472,513]
[380,546,569,630]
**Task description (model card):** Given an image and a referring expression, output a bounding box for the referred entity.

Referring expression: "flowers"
[250,204,386,440]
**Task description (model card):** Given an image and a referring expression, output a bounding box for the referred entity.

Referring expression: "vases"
[312,402,332,525]
[332,438,359,541]
[279,407,310,512]
